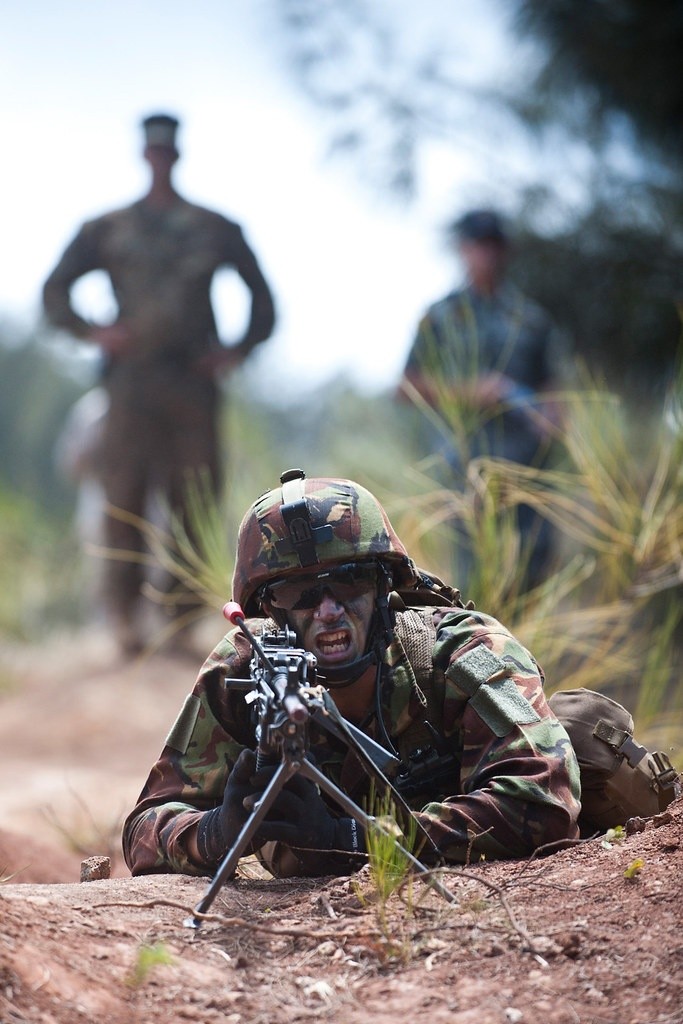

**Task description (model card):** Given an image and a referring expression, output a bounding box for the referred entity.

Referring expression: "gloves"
[197,749,284,867]
[243,764,370,874]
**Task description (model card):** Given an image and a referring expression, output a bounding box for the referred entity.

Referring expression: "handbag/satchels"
[546,685,680,832]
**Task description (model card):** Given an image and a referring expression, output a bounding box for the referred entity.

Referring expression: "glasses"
[258,560,392,609]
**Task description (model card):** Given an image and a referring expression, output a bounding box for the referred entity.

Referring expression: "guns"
[188,597,460,933]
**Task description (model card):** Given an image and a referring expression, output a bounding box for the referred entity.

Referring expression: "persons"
[42,115,275,658]
[121,468,679,879]
[399,211,570,626]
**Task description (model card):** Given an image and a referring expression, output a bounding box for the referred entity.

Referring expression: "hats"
[144,114,177,148]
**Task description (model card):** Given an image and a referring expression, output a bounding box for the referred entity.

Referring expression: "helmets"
[232,469,415,618]
